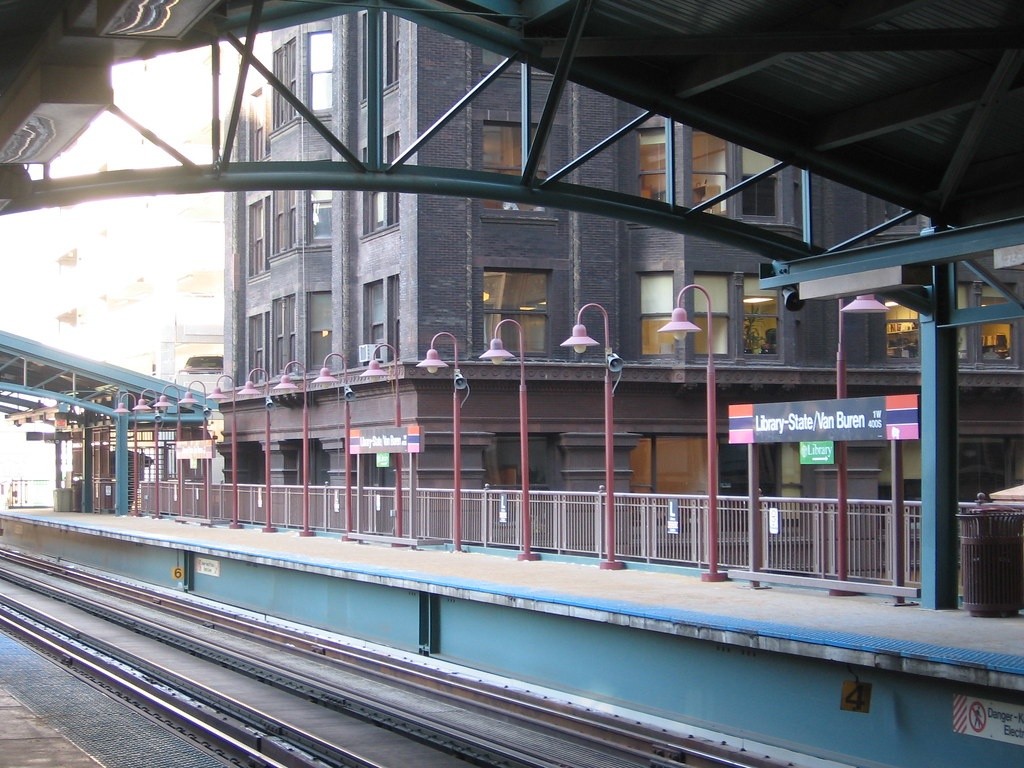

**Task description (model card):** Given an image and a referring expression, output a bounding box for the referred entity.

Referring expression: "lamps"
[781,284,805,311]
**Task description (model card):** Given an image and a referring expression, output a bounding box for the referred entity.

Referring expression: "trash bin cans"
[955,511,1024,618]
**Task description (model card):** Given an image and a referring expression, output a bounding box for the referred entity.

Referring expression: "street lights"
[824,293,893,597]
[655,283,728,583]
[132,388,163,520]
[205,374,244,529]
[359,343,410,548]
[310,353,356,542]
[113,392,142,517]
[236,367,278,532]
[558,302,627,570]
[415,332,468,552]
[272,360,317,537]
[177,380,212,519]
[152,384,187,522]
[478,318,541,562]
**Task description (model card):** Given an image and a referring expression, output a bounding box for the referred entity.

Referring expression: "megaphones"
[266,398,275,410]
[344,387,355,401]
[454,374,467,389]
[155,415,161,422]
[203,406,210,417]
[607,353,625,373]
[783,287,804,312]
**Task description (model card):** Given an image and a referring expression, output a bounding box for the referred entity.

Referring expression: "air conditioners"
[358,344,385,362]
